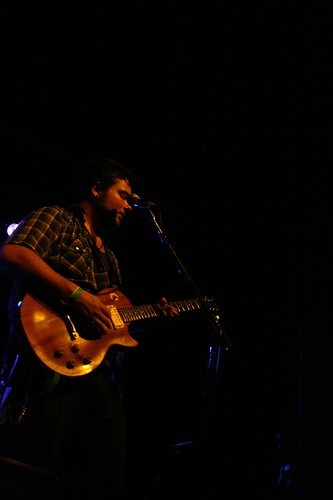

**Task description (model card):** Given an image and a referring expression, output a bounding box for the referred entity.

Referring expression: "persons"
[0,154,179,500]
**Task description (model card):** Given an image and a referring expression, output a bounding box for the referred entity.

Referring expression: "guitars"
[21,288,213,377]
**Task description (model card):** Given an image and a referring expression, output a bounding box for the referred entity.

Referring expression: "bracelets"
[66,286,83,301]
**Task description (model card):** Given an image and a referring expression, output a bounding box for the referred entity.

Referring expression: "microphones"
[126,194,155,212]
